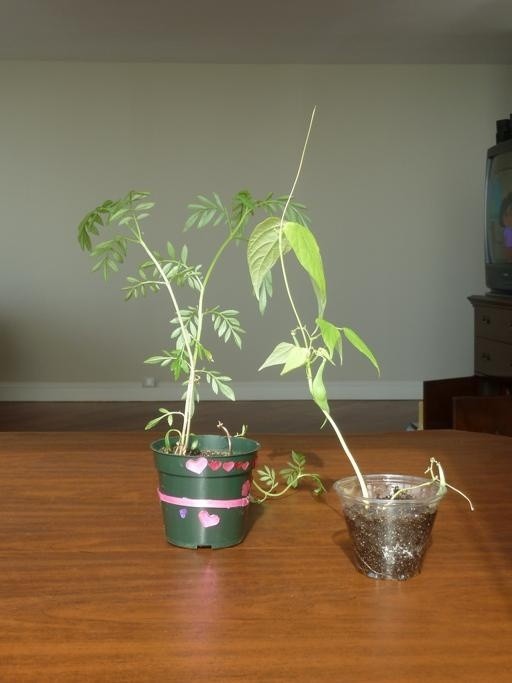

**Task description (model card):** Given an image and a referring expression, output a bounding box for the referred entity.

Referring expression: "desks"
[1,426,510,683]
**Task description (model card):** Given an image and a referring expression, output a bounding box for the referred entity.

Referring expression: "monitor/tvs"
[484,141,512,299]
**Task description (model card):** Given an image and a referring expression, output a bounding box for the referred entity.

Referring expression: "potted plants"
[75,178,325,556]
[243,95,460,586]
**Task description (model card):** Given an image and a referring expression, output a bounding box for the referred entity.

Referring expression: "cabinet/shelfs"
[466,291,511,376]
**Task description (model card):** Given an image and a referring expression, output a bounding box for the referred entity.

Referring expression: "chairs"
[418,372,511,438]
